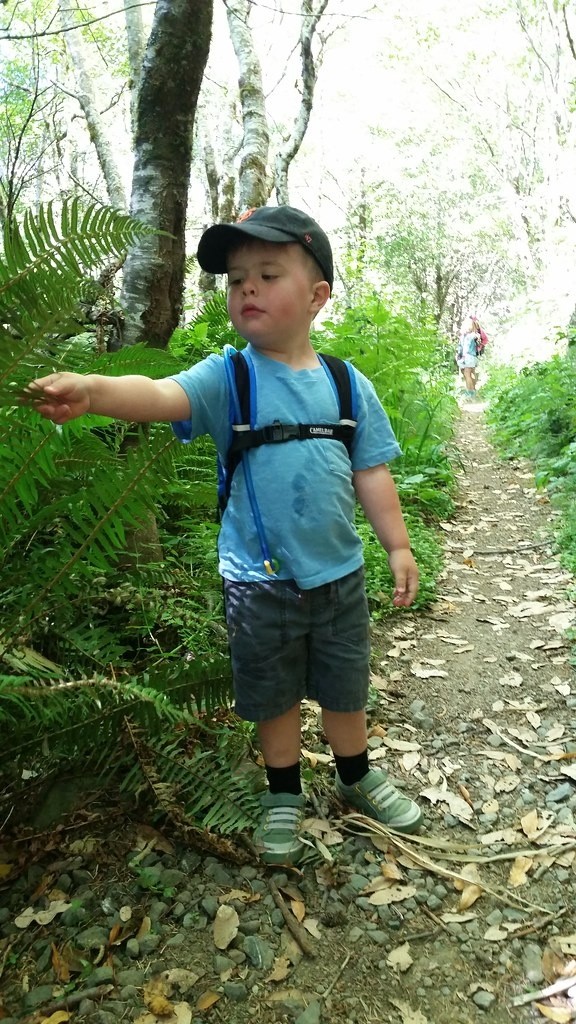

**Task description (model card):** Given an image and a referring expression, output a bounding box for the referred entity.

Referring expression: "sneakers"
[252,784,305,864]
[335,767,423,834]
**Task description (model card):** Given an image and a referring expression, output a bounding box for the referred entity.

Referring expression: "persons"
[455,314,488,398]
[19,205,424,866]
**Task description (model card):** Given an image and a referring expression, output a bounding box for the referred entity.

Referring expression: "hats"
[197,206,334,298]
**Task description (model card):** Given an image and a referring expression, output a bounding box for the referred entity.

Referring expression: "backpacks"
[475,326,489,356]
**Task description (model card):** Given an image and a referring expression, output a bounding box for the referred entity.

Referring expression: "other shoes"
[464,390,475,397]
[474,372,479,383]
